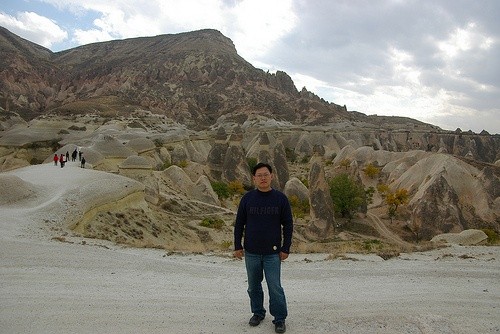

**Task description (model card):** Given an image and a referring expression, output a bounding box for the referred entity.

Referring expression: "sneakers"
[275,322,286,333]
[249,314,264,326]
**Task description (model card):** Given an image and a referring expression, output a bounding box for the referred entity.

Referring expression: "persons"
[234,162,294,334]
[54,149,85,168]
